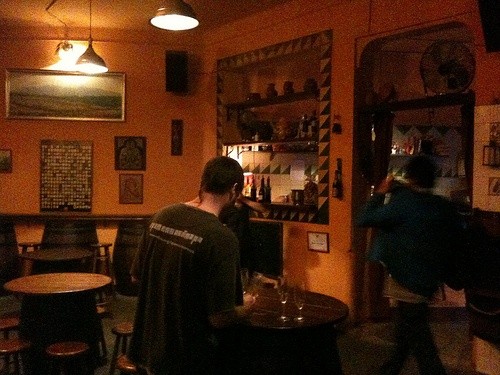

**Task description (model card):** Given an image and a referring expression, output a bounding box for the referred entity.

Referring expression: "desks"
[3,274,113,375]
[20,246,91,274]
[237,289,347,375]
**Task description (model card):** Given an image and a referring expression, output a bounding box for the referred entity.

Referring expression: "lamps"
[150,1,199,32]
[74,0,109,75]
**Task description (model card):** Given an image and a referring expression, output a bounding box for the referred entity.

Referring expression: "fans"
[421,40,476,94]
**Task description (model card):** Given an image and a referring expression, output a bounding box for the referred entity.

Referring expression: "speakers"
[165,52,187,94]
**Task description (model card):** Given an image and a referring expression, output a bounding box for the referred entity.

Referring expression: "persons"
[130,156,254,375]
[357,154,446,375]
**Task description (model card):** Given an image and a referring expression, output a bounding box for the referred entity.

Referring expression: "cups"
[249,275,264,299]
[240,267,249,294]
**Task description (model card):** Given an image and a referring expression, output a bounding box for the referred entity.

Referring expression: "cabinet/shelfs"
[224,89,323,149]
[379,91,475,201]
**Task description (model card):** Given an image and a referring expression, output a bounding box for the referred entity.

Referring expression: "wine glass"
[294,280,305,322]
[279,279,290,322]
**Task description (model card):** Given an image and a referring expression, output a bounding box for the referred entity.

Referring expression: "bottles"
[331,171,339,198]
[252,111,319,142]
[265,78,318,96]
[244,174,271,204]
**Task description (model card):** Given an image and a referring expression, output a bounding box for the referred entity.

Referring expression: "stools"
[0,239,134,375]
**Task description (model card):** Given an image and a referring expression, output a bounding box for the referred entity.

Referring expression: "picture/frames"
[0,148,13,173]
[114,135,147,171]
[119,173,144,204]
[6,68,128,121]
